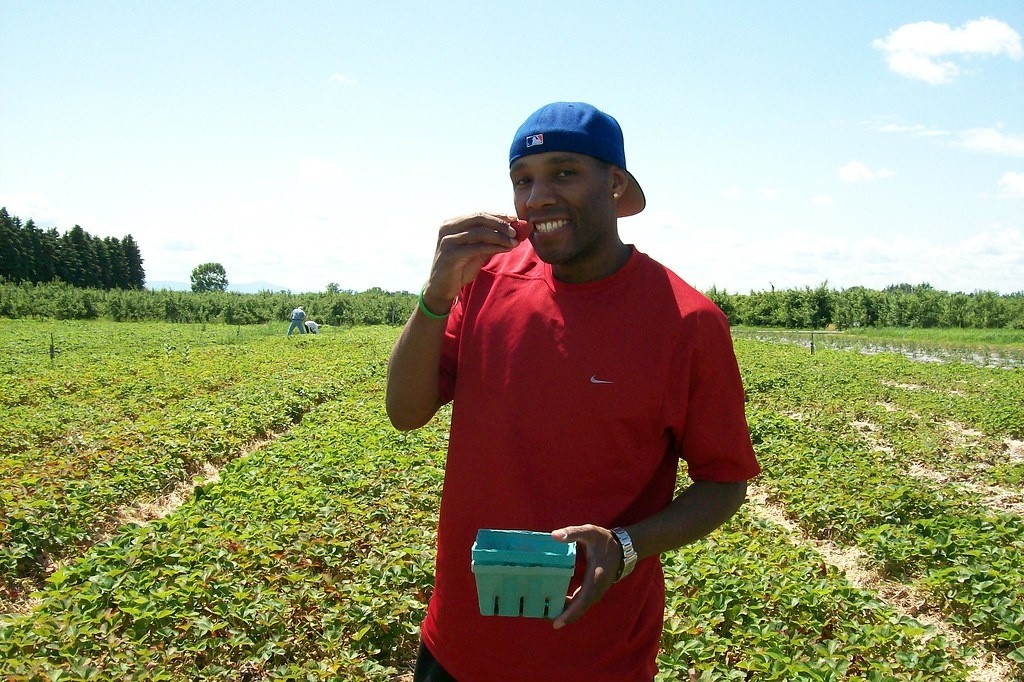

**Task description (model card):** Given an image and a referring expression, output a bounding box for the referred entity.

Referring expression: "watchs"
[610,528,637,583]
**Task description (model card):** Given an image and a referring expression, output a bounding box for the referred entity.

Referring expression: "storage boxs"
[471,529,576,619]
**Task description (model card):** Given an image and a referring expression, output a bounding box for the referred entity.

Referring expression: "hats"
[508,101,646,219]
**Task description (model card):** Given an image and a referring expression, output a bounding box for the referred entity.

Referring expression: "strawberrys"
[509,220,534,243]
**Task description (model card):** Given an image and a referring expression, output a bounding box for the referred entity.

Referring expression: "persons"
[304,321,322,334]
[385,102,762,682]
[287,307,306,335]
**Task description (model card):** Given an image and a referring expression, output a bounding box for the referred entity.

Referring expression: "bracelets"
[418,280,459,319]
